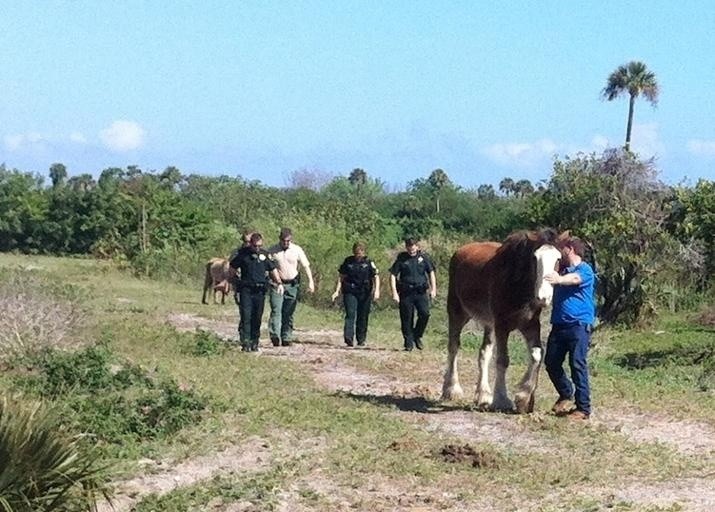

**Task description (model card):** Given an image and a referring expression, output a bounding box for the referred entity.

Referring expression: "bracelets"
[277,281,282,285]
[276,278,281,281]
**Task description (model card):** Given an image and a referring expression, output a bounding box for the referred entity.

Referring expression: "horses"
[202,257,241,305]
[442,227,573,413]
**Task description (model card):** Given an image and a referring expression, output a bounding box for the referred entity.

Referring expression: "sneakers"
[346,336,365,346]
[241,338,292,351]
[553,397,589,420]
[406,337,424,350]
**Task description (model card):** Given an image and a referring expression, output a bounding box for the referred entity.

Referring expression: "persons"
[267,228,315,346]
[388,238,436,350]
[226,226,283,346]
[217,232,285,351]
[542,235,596,421]
[331,242,379,348]
